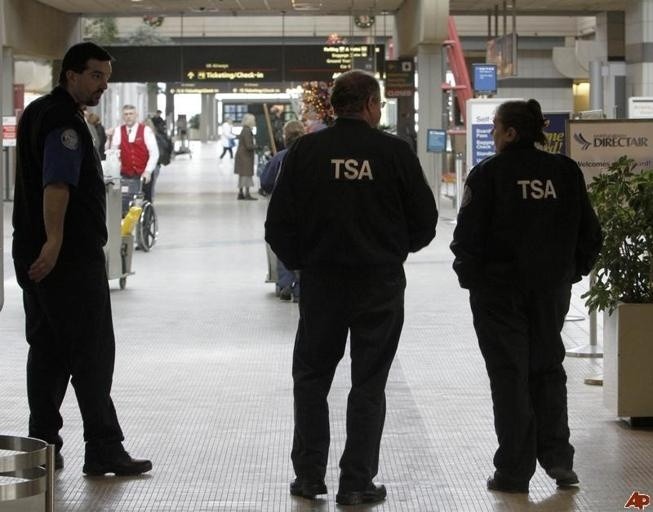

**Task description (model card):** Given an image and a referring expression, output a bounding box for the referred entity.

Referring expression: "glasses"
[374,100,386,108]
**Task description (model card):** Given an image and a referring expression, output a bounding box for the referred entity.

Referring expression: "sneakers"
[277,280,300,302]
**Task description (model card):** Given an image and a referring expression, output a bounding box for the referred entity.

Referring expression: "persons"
[234,113,262,200]
[450,99,602,493]
[260,119,305,303]
[265,69,438,505]
[83,107,107,160]
[151,110,166,128]
[219,114,237,159]
[112,104,159,251]
[13,42,152,476]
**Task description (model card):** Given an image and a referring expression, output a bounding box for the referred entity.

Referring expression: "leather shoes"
[335,485,387,506]
[289,477,328,499]
[82,445,153,475]
[548,468,580,487]
[22,451,64,480]
[237,193,259,201]
[486,475,531,493]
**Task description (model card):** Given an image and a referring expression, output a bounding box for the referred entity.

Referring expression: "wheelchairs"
[119,177,159,254]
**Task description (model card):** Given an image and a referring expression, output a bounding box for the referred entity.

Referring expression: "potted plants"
[583,154,653,430]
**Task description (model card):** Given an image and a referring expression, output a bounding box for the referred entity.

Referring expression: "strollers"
[256,144,274,197]
